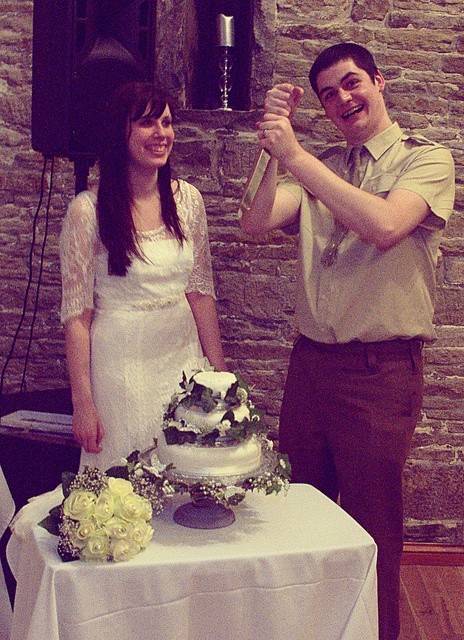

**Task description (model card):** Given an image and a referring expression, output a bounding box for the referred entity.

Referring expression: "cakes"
[156,369,262,478]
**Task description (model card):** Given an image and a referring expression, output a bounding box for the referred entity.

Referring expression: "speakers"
[31,2,151,156]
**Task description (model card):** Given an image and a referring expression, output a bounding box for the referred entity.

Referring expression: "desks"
[6,482,381,639]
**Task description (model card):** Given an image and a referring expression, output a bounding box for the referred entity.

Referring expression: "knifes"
[234,149,271,221]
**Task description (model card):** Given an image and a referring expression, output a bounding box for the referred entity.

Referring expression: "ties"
[320,143,369,268]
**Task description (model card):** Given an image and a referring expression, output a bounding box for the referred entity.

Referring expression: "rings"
[262,128,267,139]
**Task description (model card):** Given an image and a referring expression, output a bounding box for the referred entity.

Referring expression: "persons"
[60,83,230,488]
[240,42,455,640]
